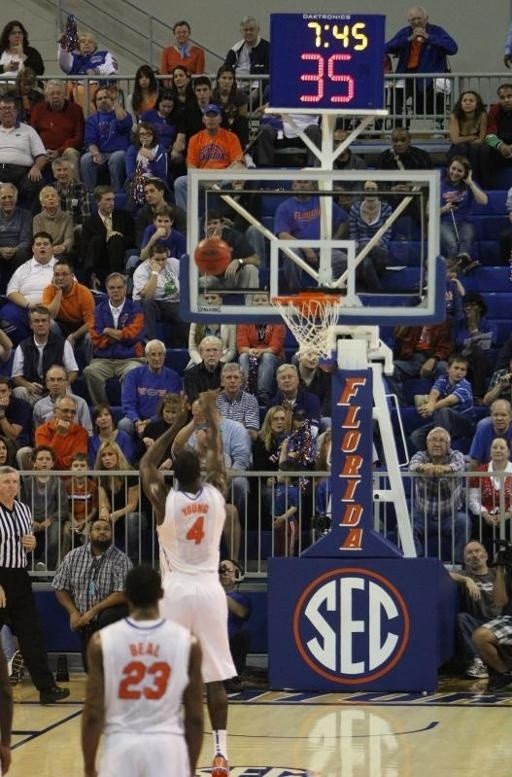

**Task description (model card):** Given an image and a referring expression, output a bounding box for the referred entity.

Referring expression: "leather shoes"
[40,685,69,703]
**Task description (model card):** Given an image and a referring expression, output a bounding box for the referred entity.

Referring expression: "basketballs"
[194,238,232,275]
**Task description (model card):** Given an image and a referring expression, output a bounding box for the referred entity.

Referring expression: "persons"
[138,388,237,777]
[1,7,510,700]
[82,566,204,776]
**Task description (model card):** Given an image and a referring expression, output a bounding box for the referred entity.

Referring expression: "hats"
[204,104,220,114]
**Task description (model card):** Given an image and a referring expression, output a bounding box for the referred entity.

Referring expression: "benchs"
[71,154,512,558]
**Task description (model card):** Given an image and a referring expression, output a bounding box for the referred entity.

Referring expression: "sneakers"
[213,754,229,777]
[489,672,512,695]
[465,658,491,678]
